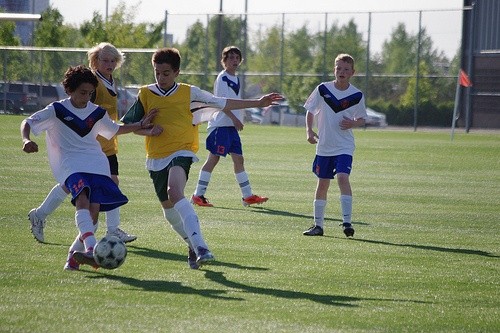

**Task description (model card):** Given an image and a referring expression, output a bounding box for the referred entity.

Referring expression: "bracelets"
[140,120,144,129]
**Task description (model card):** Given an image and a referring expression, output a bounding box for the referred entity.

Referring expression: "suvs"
[0,81,38,113]
[26,82,67,110]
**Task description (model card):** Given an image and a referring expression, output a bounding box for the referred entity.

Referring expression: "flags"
[460,72,472,86]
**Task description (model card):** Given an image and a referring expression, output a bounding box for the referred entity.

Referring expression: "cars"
[367,109,387,127]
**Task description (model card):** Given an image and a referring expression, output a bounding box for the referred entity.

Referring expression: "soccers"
[92,234,128,269]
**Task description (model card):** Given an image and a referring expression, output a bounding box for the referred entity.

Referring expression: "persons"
[190,46,268,207]
[28,43,137,243]
[303,55,366,235]
[120,48,283,269]
[21,65,160,270]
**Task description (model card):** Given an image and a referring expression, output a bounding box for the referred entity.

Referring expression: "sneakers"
[72,247,100,268]
[27,208,44,243]
[190,194,214,208]
[105,229,137,243]
[195,247,214,266]
[64,251,81,271]
[339,222,354,236]
[188,250,202,269]
[242,195,269,207]
[301,225,325,237]
